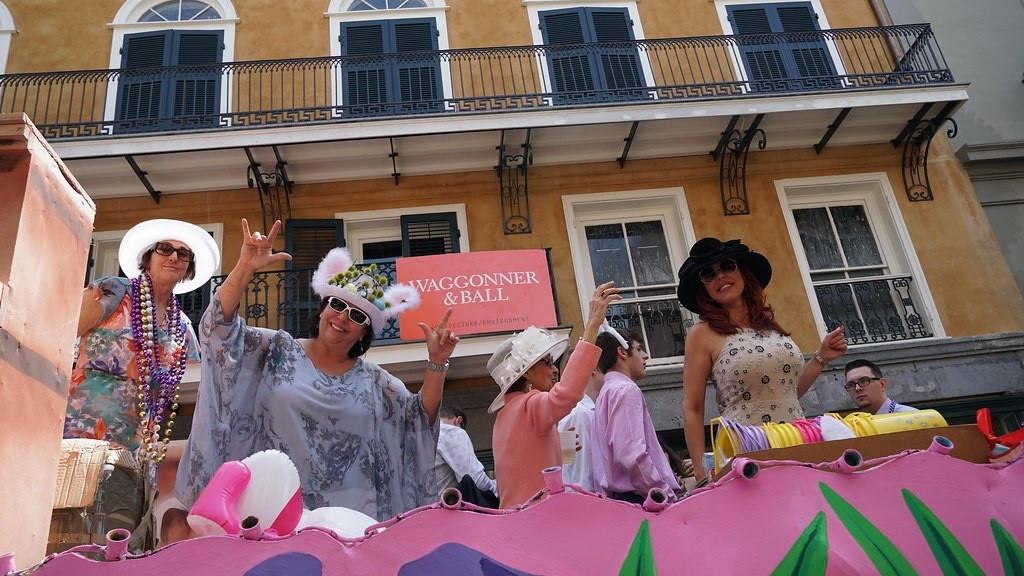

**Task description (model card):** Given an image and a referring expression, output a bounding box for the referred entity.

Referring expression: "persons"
[61,218,219,486]
[557,348,605,492]
[487,281,622,509]
[844,360,919,415]
[682,452,714,476]
[590,328,681,504]
[436,400,499,501]
[675,237,847,488]
[172,218,459,530]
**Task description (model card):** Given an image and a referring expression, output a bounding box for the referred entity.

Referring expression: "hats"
[118,219,219,295]
[677,237,772,314]
[313,248,421,335]
[487,327,570,414]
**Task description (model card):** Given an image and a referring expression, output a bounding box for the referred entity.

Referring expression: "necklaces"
[131,273,187,464]
[889,400,897,413]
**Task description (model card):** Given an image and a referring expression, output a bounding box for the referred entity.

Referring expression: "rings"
[601,291,606,298]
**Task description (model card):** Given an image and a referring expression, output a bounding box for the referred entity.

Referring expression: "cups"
[559,431,576,464]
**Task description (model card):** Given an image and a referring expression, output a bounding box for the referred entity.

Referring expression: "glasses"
[699,259,736,284]
[540,356,555,367]
[844,376,879,392]
[328,297,371,325]
[151,242,195,262]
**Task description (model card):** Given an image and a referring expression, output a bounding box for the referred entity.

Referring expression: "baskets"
[53,438,109,509]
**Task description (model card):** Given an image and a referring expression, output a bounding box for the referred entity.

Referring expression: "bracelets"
[427,359,449,371]
[814,350,829,367]
[695,477,707,487]
[579,337,585,341]
